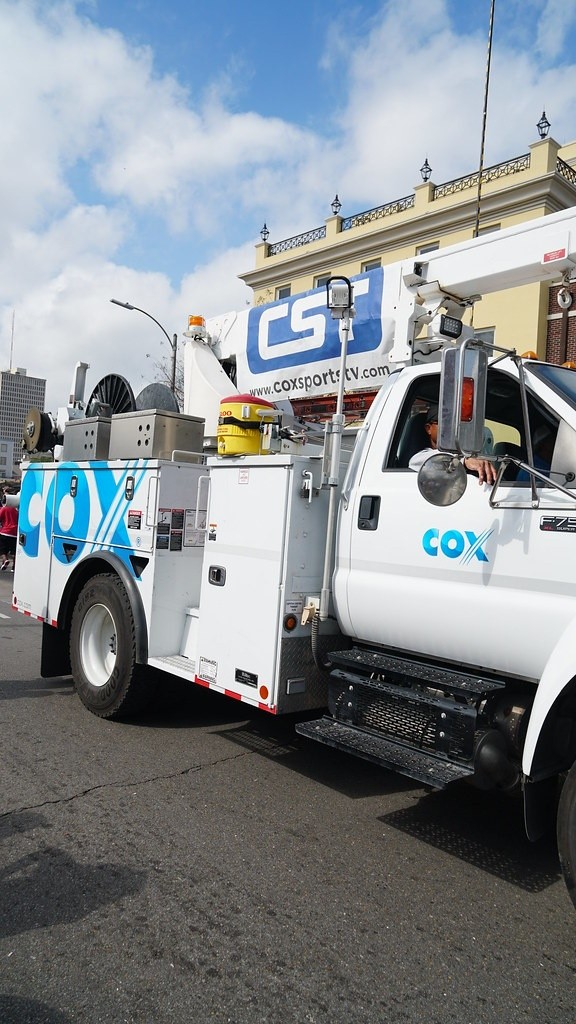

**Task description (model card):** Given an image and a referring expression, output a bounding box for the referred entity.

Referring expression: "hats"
[425,404,451,424]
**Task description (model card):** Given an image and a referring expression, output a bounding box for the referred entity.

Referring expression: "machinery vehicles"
[9,208,576,913]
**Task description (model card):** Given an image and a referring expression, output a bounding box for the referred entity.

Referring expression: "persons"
[0,486,20,573]
[516,423,557,481]
[409,404,498,485]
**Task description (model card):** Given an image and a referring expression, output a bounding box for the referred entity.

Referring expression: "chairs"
[399,413,433,464]
[492,442,527,481]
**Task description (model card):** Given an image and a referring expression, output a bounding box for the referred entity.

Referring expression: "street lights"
[109,296,179,393]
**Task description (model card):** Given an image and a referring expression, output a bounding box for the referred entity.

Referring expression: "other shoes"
[10,566,14,572]
[1,559,10,570]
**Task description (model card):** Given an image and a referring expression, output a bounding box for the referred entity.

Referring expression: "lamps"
[536,111,551,139]
[261,224,269,242]
[331,195,342,215]
[420,158,432,182]
